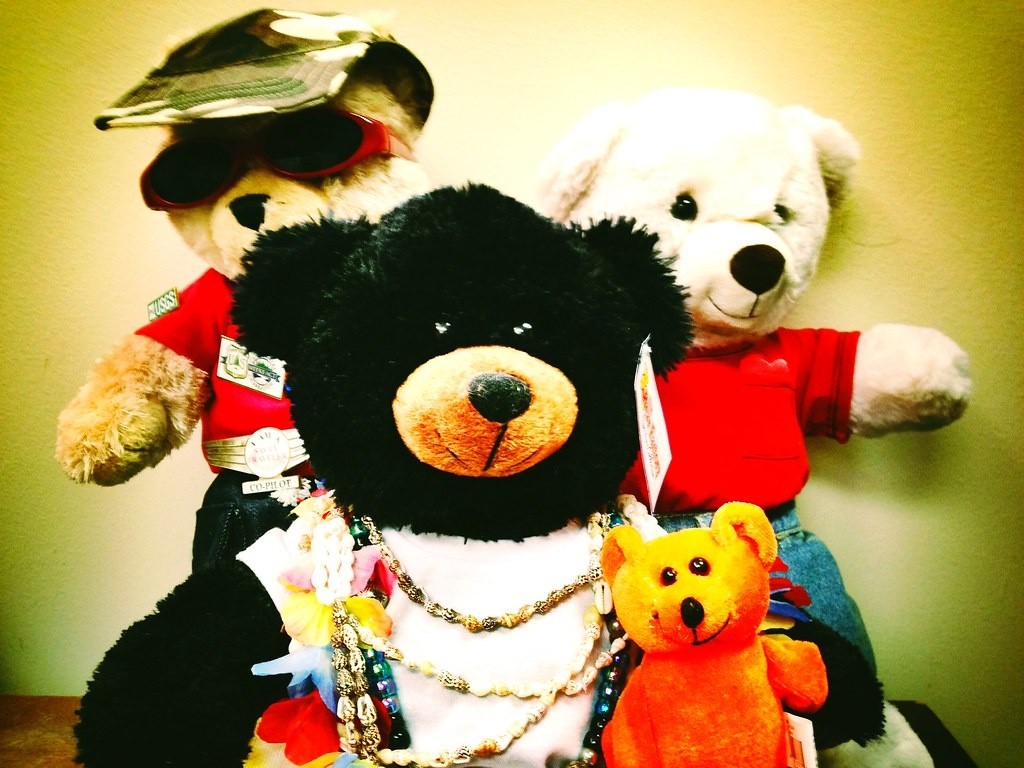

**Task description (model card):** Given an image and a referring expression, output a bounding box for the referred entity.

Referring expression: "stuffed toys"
[537,89,968,768]
[73,182,691,768]
[58,11,435,579]
[602,502,828,768]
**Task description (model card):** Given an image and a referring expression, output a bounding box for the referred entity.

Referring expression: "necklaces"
[332,516,628,768]
